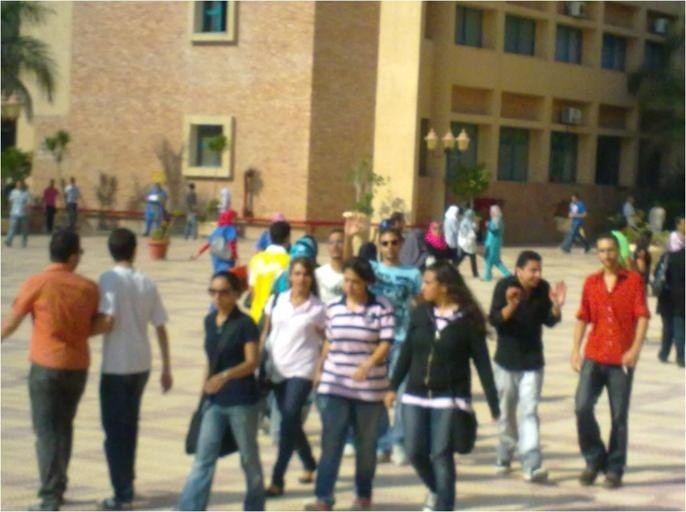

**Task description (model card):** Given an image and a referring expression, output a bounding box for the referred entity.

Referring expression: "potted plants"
[145,219,175,261]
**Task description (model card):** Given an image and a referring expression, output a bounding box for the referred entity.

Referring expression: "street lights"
[424,129,470,212]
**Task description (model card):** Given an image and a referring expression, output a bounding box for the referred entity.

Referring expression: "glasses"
[380,240,397,246]
[208,288,229,296]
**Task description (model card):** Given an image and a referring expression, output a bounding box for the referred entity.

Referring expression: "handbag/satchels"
[185,411,238,457]
[258,291,280,390]
[651,252,670,297]
[209,225,233,262]
[450,408,477,454]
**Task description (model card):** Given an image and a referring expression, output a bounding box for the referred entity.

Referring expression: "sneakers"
[602,470,623,489]
[522,466,548,482]
[264,443,408,511]
[27,502,59,511]
[421,490,440,512]
[101,496,133,510]
[579,468,599,486]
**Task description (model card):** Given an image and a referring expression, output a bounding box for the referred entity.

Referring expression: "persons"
[559,192,591,252]
[175,271,266,511]
[38,177,62,235]
[4,178,34,249]
[571,232,650,487]
[248,211,435,458]
[383,259,500,508]
[622,196,686,365]
[88,227,176,511]
[490,251,568,480]
[185,183,199,240]
[304,257,395,508]
[427,204,513,281]
[139,181,168,237]
[0,226,100,512]
[217,189,231,212]
[58,177,85,228]
[260,256,328,497]
